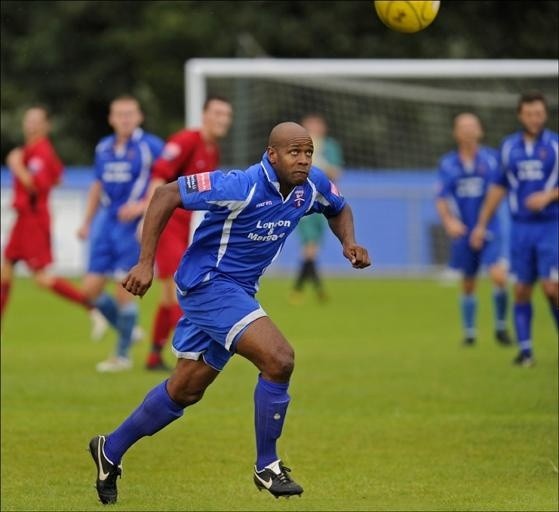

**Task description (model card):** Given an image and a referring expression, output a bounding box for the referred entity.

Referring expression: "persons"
[435,112,514,346]
[78,95,166,371]
[89,121,371,505]
[470,91,559,367]
[135,97,234,371]
[0,105,108,344]
[292,115,344,307]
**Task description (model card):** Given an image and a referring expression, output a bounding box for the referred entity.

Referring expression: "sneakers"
[254,460,303,497]
[90,435,122,505]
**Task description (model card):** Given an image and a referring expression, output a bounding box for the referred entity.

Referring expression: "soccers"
[375,0,441,33]
